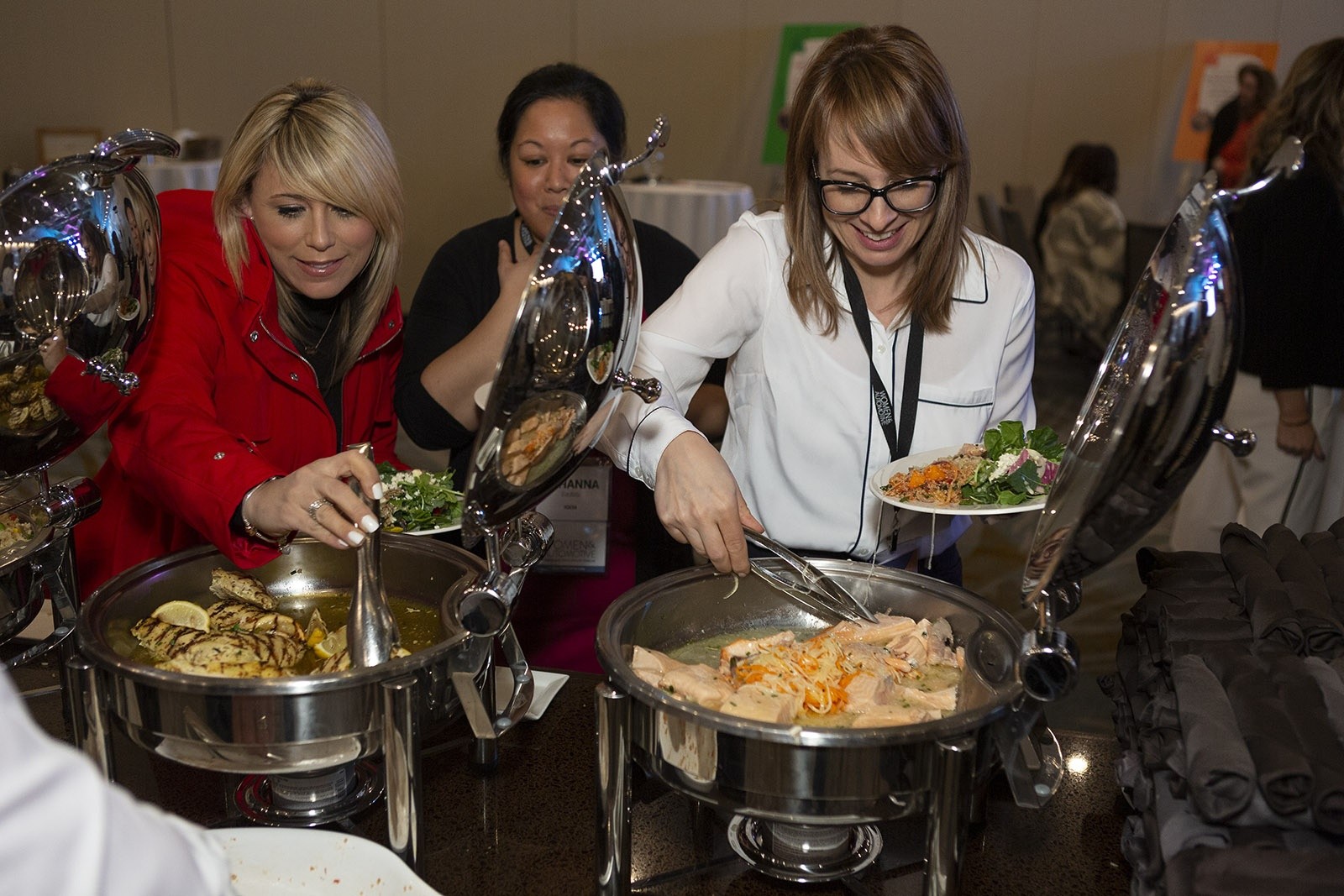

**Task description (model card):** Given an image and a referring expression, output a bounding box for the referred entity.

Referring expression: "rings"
[44,345,47,349]
[308,499,333,519]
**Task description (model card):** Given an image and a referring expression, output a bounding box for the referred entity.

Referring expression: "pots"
[495,389,588,494]
[0,346,86,438]
[0,463,76,673]
[594,559,1064,827]
[75,531,537,774]
[14,318,74,345]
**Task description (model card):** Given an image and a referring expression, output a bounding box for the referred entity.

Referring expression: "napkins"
[1096,520,1344,896]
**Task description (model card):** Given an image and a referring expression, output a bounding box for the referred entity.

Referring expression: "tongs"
[739,522,879,628]
[346,442,400,668]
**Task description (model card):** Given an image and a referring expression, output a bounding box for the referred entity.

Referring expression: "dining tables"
[6,660,1134,896]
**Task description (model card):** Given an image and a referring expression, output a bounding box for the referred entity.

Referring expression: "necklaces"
[299,304,337,356]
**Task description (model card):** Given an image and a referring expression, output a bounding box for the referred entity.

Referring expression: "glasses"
[811,158,950,215]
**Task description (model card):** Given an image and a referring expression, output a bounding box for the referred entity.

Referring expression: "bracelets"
[1279,419,1309,426]
[242,476,292,555]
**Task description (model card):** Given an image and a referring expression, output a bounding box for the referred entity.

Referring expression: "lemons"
[151,600,210,635]
[307,609,348,656]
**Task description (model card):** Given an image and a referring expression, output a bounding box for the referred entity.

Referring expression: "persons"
[392,61,729,678]
[38,330,146,439]
[1034,142,1126,331]
[594,26,1037,587]
[1170,37,1344,555]
[571,183,643,456]
[73,79,415,603]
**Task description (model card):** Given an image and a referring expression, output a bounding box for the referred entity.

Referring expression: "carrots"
[736,642,860,714]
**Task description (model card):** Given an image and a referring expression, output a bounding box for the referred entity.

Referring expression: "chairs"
[978,182,1075,355]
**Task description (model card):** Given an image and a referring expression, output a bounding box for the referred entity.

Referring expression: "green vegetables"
[375,460,464,533]
[960,420,1068,508]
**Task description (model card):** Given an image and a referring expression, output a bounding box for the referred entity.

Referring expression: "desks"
[616,180,754,260]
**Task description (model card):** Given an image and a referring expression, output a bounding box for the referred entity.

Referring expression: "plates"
[115,297,141,321]
[587,345,614,384]
[11,598,55,646]
[493,665,570,721]
[402,488,467,536]
[869,446,1048,514]
